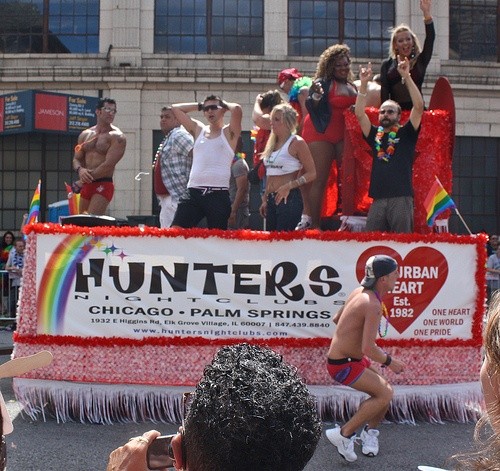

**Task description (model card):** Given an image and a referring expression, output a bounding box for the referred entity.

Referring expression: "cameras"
[146,434,176,470]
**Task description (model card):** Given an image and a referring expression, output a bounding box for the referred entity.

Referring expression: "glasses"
[490,238,498,240]
[377,110,400,116]
[100,107,117,114]
[203,105,223,112]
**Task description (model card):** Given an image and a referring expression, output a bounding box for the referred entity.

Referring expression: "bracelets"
[381,355,392,368]
[424,17,432,21]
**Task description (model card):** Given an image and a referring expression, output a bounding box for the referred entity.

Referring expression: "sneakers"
[326,424,360,462]
[361,423,380,456]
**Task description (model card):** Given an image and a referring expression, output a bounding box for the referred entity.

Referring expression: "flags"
[424,179,455,227]
[24,185,41,225]
[65,186,80,215]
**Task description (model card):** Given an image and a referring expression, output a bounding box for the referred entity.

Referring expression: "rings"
[139,438,148,443]
[129,438,138,442]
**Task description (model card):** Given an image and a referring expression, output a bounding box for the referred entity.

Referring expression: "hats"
[360,254,398,287]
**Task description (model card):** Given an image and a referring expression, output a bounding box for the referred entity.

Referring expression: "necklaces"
[371,287,388,337]
[375,126,398,162]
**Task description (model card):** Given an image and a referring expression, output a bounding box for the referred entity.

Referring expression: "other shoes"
[294,220,312,230]
[5,323,15,331]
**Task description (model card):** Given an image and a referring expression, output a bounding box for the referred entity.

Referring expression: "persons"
[379,0,436,112]
[72,98,126,215]
[485,233,500,293]
[0,232,25,331]
[153,95,251,231]
[325,255,406,463]
[254,44,425,235]
[107,344,322,471]
[452,298,500,471]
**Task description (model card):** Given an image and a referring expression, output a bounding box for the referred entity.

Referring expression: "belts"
[191,187,229,196]
